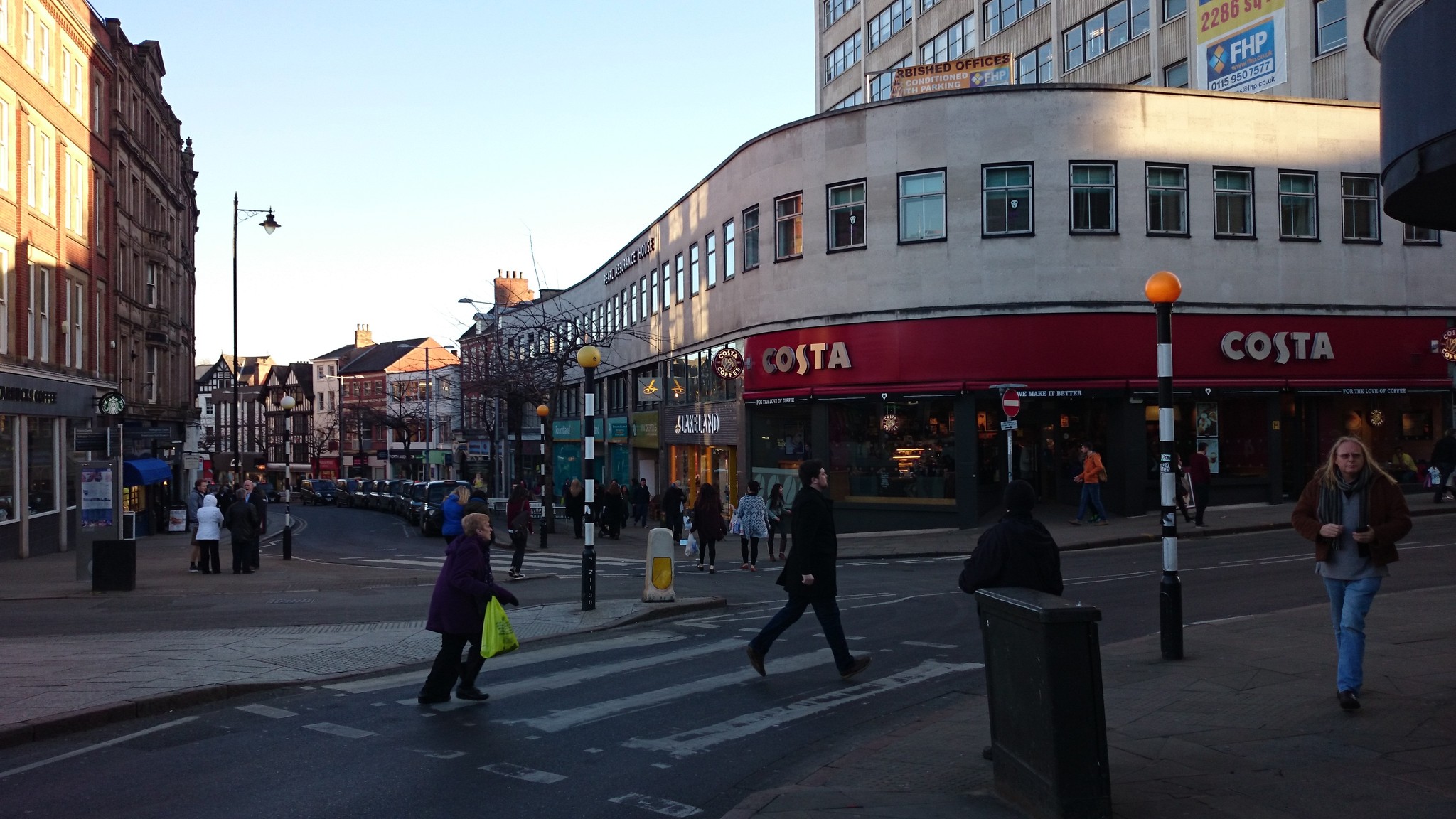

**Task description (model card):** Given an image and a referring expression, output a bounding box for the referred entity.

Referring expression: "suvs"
[336,478,473,535]
[299,479,337,506]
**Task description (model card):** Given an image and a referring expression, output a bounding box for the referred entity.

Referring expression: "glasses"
[1336,454,1364,460]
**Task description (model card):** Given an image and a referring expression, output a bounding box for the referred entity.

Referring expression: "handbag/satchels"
[1423,467,1441,488]
[1446,470,1456,490]
[516,498,529,531]
[685,532,700,556]
[716,515,727,542]
[683,516,692,530]
[729,509,744,535]
[431,499,446,528]
[480,596,519,658]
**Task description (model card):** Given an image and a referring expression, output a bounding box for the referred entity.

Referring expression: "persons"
[189,478,268,574]
[1392,429,1456,504]
[441,473,790,571]
[746,461,872,680]
[959,479,1065,596]
[473,474,484,491]
[417,512,518,703]
[849,443,1212,528]
[790,434,813,462]
[520,471,532,491]
[1292,435,1412,709]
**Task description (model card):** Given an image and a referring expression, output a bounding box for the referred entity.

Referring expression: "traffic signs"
[1001,420,1018,431]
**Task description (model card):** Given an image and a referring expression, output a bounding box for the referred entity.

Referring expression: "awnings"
[124,458,172,488]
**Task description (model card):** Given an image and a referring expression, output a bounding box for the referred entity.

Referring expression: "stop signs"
[1002,389,1020,417]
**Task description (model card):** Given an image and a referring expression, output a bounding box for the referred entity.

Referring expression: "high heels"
[709,569,715,574]
[697,564,704,571]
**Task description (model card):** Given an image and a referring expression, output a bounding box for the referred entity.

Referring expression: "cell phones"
[1357,525,1369,533]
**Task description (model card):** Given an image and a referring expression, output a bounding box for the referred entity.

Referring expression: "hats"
[674,480,683,489]
[1005,480,1034,511]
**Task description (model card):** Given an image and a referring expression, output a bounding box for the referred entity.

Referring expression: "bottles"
[739,527,744,535]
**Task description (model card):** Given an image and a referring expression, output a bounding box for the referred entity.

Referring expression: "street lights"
[458,298,535,515]
[280,396,295,560]
[574,346,600,608]
[398,344,455,482]
[1145,272,1182,662]
[536,405,549,552]
[232,188,281,499]
[320,374,364,480]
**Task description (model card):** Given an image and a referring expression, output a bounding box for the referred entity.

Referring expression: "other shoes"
[1068,519,1082,525]
[614,533,619,540]
[1337,689,1360,709]
[839,656,871,679]
[418,689,452,704]
[1440,500,1446,504]
[1196,523,1208,527]
[745,646,766,677]
[452,689,489,701]
[243,570,255,574]
[188,565,199,572]
[514,573,526,579]
[1085,516,1100,523]
[508,568,515,577]
[672,535,681,542]
[1186,519,1196,522]
[750,566,757,571]
[644,525,648,528]
[740,565,748,570]
[1093,520,1108,526]
[779,553,787,560]
[769,554,776,561]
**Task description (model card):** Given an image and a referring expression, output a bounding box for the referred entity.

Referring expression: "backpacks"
[1092,453,1107,482]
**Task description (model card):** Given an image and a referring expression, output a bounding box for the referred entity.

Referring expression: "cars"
[255,482,279,503]
